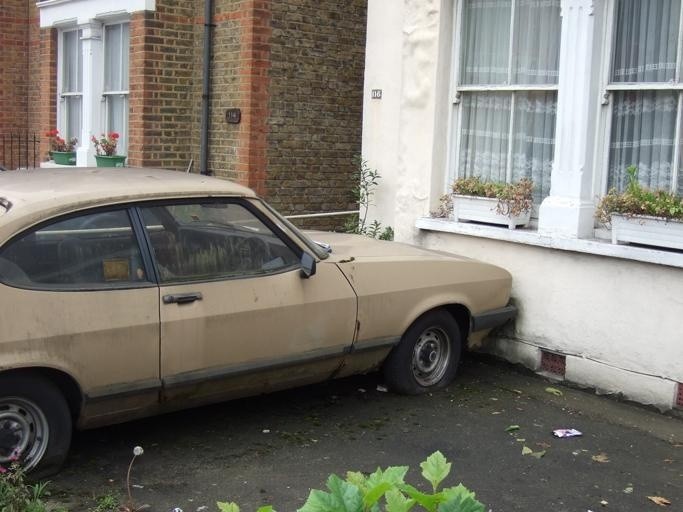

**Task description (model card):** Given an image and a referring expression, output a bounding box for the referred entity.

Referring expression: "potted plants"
[593,164,683,254]
[429,175,536,230]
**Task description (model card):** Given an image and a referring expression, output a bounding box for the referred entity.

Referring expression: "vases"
[95,155,126,169]
[52,152,76,165]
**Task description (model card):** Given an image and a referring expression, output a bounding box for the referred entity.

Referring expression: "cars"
[0,166,518,485]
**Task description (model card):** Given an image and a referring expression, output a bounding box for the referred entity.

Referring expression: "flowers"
[44,130,79,152]
[90,132,124,155]
